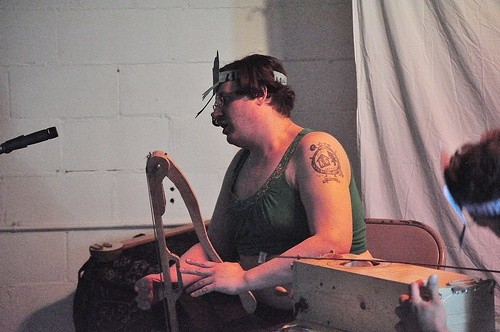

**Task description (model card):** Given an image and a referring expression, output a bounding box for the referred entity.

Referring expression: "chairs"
[357,217,447,274]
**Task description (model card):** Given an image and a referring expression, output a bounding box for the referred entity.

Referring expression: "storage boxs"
[290,252,497,332]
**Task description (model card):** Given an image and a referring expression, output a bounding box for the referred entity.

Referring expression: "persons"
[394,126,500,332]
[133,55,372,332]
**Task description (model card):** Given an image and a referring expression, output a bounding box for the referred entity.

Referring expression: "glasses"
[213,89,250,111]
